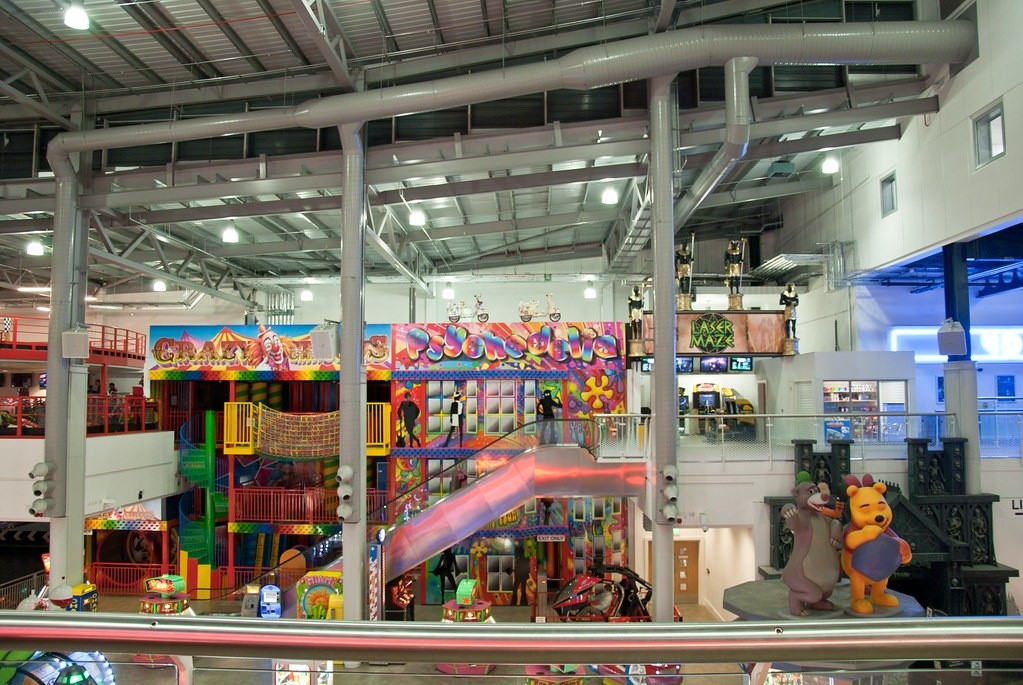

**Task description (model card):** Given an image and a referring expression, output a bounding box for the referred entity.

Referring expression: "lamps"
[817,149,842,174]
[299,282,312,299]
[407,202,426,226]
[222,217,240,243]
[600,182,618,205]
[441,282,455,298]
[65,1,92,30]
[27,231,45,256]
[582,279,596,300]
[153,276,168,290]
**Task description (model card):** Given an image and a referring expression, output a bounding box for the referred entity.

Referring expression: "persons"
[724,238,744,294]
[617,571,636,617]
[592,548,603,575]
[571,585,612,622]
[396,392,420,447]
[674,241,693,293]
[627,286,643,339]
[509,547,530,605]
[779,279,799,338]
[88,379,116,397]
[442,391,463,448]
[432,548,460,604]
[535,390,564,445]
